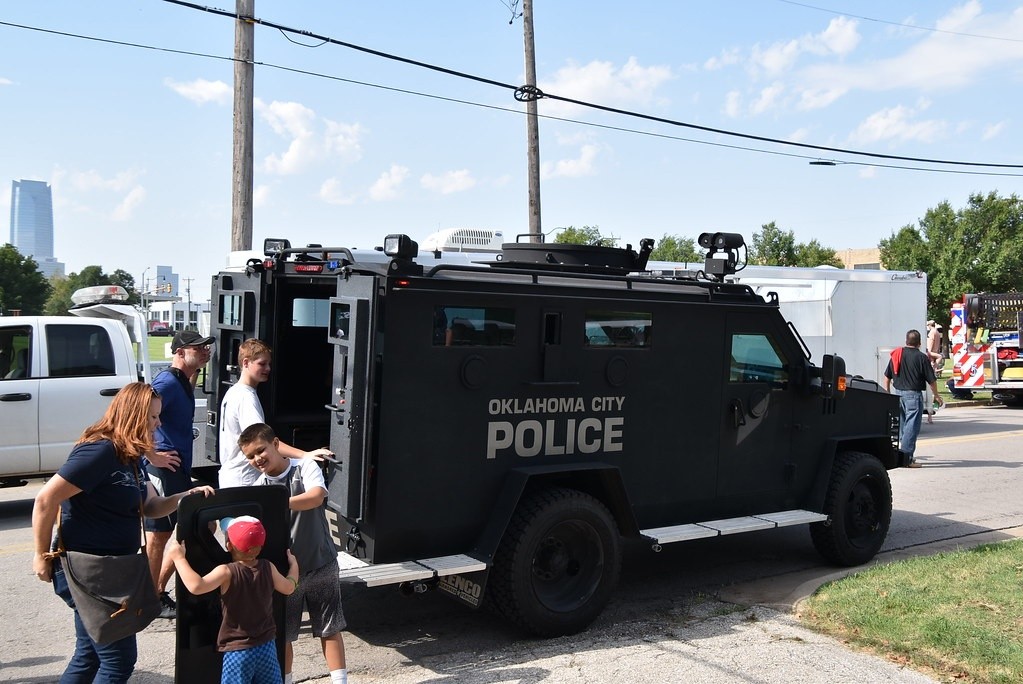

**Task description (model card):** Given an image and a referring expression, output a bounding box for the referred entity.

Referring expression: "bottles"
[933,401,939,411]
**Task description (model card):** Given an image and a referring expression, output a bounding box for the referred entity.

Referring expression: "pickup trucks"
[0,286,220,488]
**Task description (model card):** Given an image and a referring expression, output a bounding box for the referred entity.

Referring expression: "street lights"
[146,274,166,293]
[141,267,150,307]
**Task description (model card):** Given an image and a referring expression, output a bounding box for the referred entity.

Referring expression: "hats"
[172,330,216,354]
[220,517,265,553]
[927,319,935,326]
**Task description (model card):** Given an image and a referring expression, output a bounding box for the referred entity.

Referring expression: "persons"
[169,515,299,684]
[32,382,213,684]
[884,330,943,468]
[218,338,335,490]
[139,331,216,617]
[238,423,348,684]
[926,320,942,423]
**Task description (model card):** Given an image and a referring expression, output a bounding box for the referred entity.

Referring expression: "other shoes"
[964,393,974,401]
[160,591,177,609]
[907,461,921,468]
[160,603,176,618]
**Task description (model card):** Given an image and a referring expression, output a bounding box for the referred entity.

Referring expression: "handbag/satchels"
[59,549,162,646]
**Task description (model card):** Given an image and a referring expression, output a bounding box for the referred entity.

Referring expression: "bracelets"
[287,575,299,591]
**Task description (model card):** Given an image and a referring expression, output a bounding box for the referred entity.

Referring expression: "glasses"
[149,387,158,399]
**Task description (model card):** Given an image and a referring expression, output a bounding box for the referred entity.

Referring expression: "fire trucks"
[953,290,1023,406]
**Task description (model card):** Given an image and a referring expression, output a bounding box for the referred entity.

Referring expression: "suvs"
[203,229,915,641]
